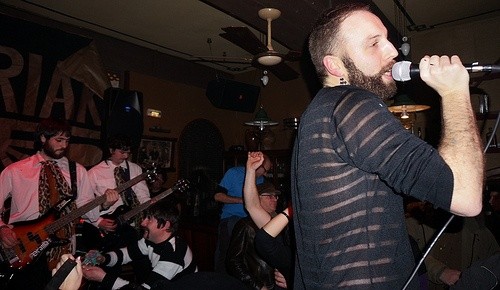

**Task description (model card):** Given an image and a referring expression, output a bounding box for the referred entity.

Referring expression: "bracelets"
[101,202,110,210]
[280,211,290,222]
[0,225,9,231]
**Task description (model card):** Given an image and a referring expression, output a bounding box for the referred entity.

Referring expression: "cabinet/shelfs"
[262,149,291,203]
[177,223,218,273]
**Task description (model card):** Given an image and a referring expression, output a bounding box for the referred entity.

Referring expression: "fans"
[194,7,309,114]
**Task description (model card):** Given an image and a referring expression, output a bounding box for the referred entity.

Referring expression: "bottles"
[486,127,497,147]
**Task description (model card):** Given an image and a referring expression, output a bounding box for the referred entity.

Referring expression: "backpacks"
[101,87,144,139]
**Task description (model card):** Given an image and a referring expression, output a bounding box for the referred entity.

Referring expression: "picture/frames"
[476,114,500,154]
[132,136,177,174]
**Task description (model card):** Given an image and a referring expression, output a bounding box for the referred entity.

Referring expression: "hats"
[256,183,281,195]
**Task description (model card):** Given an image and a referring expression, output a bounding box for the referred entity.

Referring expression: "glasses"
[262,165,268,174]
[261,194,278,200]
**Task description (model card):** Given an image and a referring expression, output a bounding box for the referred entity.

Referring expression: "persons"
[0,118,292,290]
[405,177,500,290]
[291,3,484,290]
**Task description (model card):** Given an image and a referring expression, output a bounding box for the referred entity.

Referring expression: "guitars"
[0,164,160,281]
[82,179,192,251]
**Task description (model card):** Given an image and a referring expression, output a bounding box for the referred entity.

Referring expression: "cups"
[478,94,489,113]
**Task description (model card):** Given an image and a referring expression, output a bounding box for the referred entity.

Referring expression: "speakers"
[101,87,143,139]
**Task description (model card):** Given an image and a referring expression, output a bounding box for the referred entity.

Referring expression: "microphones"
[391,60,500,82]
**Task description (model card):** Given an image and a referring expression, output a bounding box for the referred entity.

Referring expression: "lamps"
[243,92,279,131]
[387,10,431,115]
